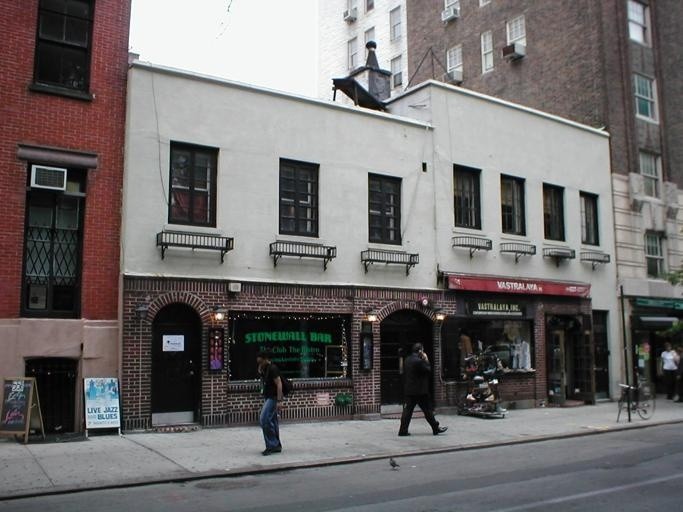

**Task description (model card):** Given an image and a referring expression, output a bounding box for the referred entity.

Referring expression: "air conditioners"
[30,164,68,192]
[502,42,525,60]
[442,70,462,84]
[441,7,457,23]
[344,10,356,21]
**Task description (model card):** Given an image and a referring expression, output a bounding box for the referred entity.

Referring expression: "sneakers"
[262,444,284,455]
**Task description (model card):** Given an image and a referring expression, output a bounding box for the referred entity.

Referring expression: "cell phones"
[418,350,424,355]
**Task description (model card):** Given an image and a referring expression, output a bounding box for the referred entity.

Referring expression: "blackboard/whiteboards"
[0,376,35,434]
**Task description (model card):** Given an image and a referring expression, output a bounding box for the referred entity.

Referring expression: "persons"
[255,350,284,455]
[512,336,532,370]
[674,346,683,403]
[660,341,680,399]
[457,327,472,367]
[398,342,448,435]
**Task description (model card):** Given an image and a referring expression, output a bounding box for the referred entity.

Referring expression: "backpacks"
[276,369,296,398]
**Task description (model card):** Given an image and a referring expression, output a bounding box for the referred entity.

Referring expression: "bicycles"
[616,378,655,423]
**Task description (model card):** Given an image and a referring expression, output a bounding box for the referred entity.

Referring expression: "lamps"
[434,307,446,325]
[365,305,378,321]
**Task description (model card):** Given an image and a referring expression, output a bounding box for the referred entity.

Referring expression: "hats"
[256,351,270,359]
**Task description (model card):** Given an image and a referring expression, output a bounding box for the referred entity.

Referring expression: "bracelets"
[276,400,283,402]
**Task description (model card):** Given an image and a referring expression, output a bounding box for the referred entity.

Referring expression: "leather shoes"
[433,427,447,435]
[397,432,411,437]
